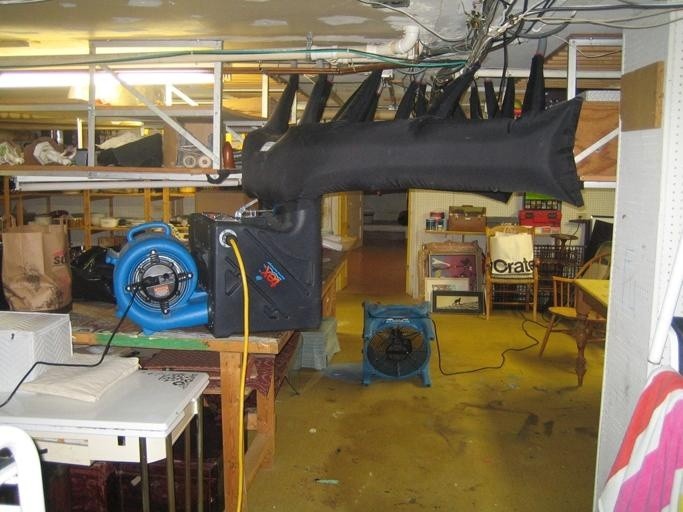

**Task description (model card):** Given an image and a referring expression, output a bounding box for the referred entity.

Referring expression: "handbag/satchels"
[0,224,73,313]
[489,230,534,279]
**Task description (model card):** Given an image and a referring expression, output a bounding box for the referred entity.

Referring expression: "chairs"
[482,223,610,387]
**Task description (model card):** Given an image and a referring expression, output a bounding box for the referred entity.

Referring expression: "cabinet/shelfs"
[1,175,195,245]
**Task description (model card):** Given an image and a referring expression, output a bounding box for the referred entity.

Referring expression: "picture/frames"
[418,240,482,314]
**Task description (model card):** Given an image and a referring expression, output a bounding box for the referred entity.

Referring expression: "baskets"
[524,199,558,210]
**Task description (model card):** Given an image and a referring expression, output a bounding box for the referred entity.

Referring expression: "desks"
[2,245,346,512]
